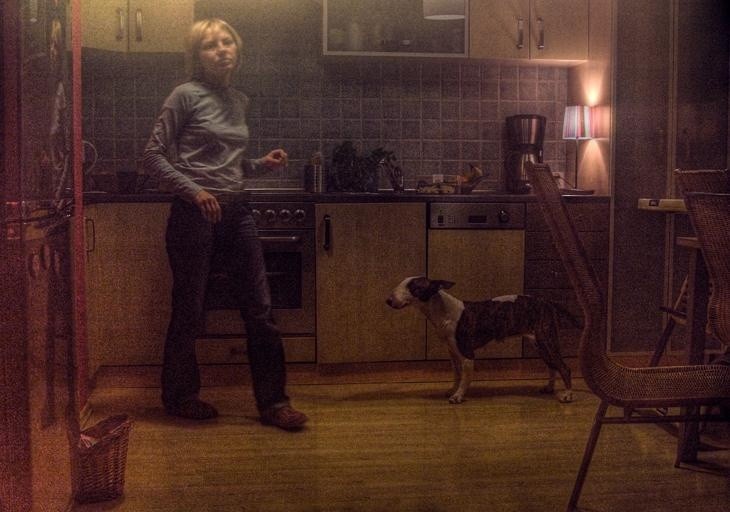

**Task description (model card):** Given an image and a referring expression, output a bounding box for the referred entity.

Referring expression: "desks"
[314,202,426,363]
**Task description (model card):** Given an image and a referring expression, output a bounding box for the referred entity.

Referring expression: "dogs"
[384,275,583,405]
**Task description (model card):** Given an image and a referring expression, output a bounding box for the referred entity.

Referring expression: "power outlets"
[552,172,564,189]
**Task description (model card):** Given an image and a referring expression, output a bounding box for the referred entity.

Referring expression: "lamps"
[560,105,593,195]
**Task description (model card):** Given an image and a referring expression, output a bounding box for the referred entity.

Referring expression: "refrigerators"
[0,0,82,512]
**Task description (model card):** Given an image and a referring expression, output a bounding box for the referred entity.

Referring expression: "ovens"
[180,198,319,365]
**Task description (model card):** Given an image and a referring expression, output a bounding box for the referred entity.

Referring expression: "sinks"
[340,189,383,197]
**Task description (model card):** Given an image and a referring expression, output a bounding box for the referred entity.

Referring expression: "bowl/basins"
[92,172,152,195]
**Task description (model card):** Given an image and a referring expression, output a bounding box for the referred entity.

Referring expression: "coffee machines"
[504,113,546,194]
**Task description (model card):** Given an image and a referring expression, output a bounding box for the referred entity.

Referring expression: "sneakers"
[262,406,306,427]
[164,398,214,420]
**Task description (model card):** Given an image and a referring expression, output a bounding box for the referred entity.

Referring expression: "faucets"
[378,159,406,193]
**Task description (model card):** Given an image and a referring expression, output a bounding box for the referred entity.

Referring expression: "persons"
[140,18,310,430]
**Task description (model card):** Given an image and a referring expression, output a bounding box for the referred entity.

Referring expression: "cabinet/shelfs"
[566,0,729,357]
[84,205,100,390]
[464,0,589,68]
[523,202,610,358]
[82,0,194,56]
